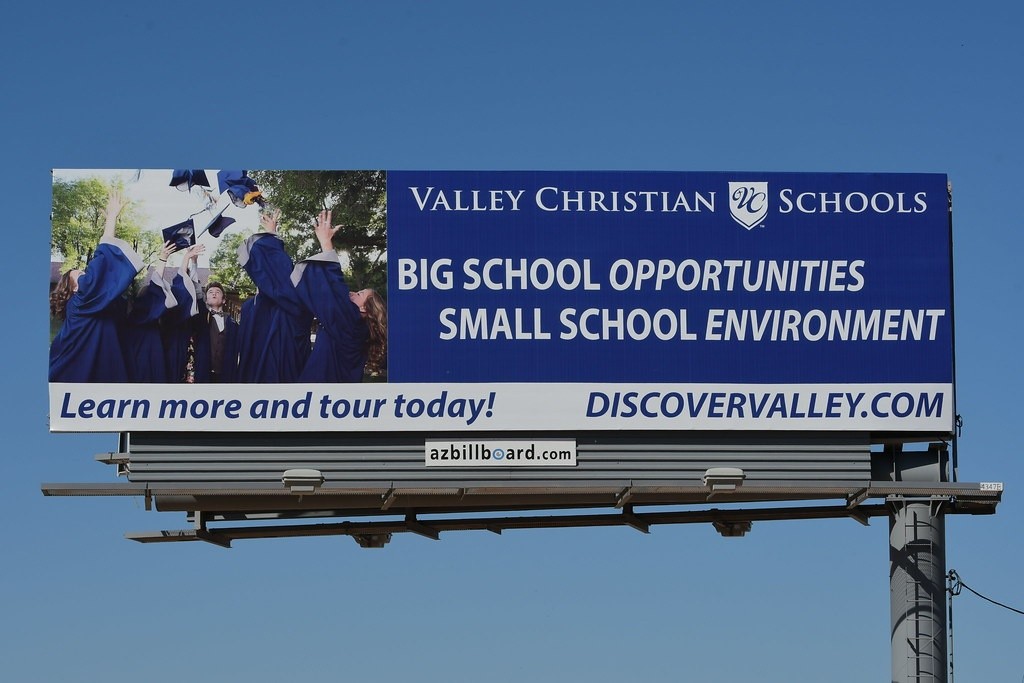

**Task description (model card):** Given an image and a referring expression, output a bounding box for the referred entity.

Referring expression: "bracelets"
[158,257,168,263]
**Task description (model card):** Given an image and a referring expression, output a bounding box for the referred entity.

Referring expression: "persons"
[50,189,386,384]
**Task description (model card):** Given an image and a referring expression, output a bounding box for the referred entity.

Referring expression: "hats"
[161,204,214,254]
[169,169,218,205]
[217,170,268,209]
[197,203,236,238]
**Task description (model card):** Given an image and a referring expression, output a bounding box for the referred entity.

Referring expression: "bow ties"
[211,309,224,317]
